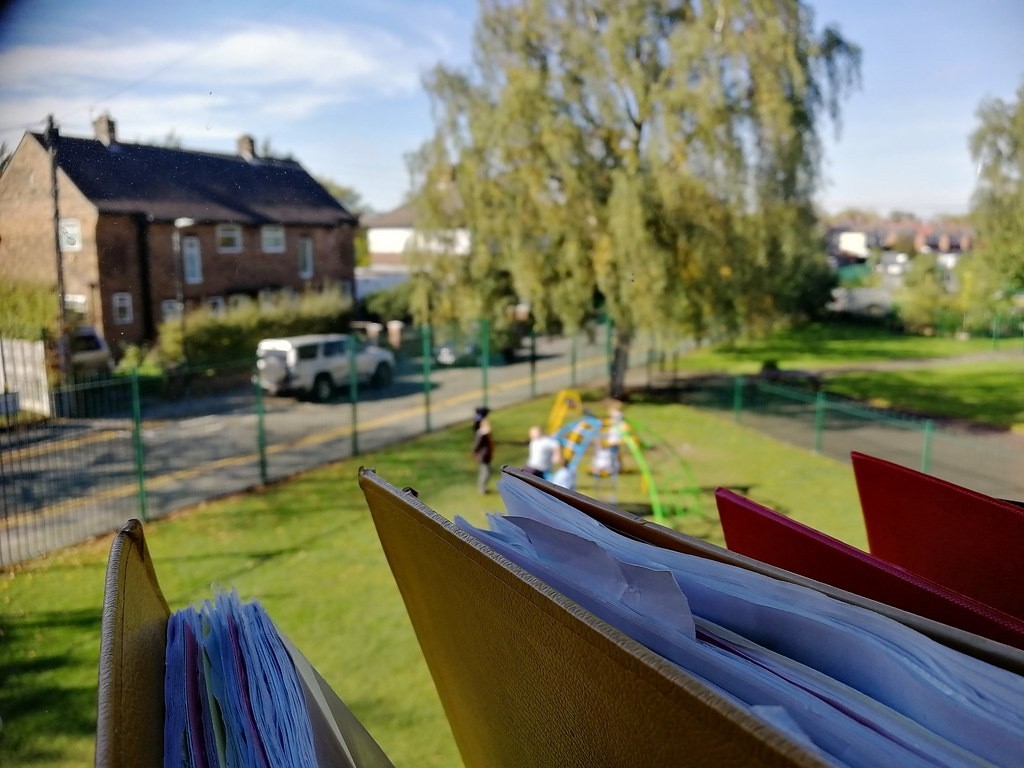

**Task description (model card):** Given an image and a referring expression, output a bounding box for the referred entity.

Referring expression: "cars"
[430,336,517,369]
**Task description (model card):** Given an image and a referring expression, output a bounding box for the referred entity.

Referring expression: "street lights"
[173,218,198,366]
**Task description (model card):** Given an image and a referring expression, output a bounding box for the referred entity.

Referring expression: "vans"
[69,329,114,381]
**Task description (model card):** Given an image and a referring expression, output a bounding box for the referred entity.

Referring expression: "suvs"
[252,333,397,403]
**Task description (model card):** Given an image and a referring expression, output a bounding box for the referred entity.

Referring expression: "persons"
[524,425,575,491]
[473,408,494,494]
